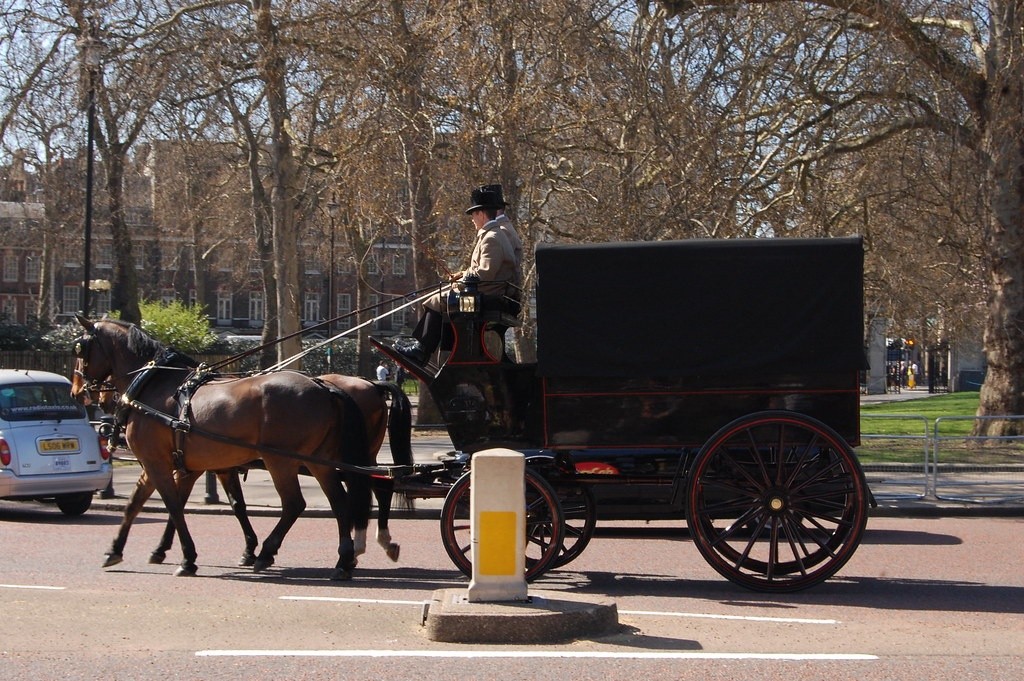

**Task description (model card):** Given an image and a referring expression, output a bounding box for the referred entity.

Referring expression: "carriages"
[70,234,879,596]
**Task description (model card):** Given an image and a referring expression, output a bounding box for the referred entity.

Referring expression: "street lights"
[74,18,109,320]
[326,190,340,344]
[82,278,111,317]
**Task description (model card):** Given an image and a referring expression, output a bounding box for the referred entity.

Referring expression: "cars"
[0,369,113,519]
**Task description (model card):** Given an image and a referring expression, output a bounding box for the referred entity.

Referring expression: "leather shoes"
[391,341,428,368]
[395,339,415,347]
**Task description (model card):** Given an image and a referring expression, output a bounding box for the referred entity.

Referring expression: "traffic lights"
[906,338,914,346]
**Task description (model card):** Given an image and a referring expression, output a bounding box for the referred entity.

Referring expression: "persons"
[395,361,404,388]
[887,361,921,391]
[385,364,393,400]
[392,185,524,369]
[377,361,389,381]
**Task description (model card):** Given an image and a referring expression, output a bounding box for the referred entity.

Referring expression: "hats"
[480,184,511,206]
[465,189,503,214]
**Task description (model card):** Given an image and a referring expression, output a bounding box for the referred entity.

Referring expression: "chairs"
[438,274,525,329]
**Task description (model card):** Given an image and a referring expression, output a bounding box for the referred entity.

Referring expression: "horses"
[70,312,369,580]
[98,362,413,568]
